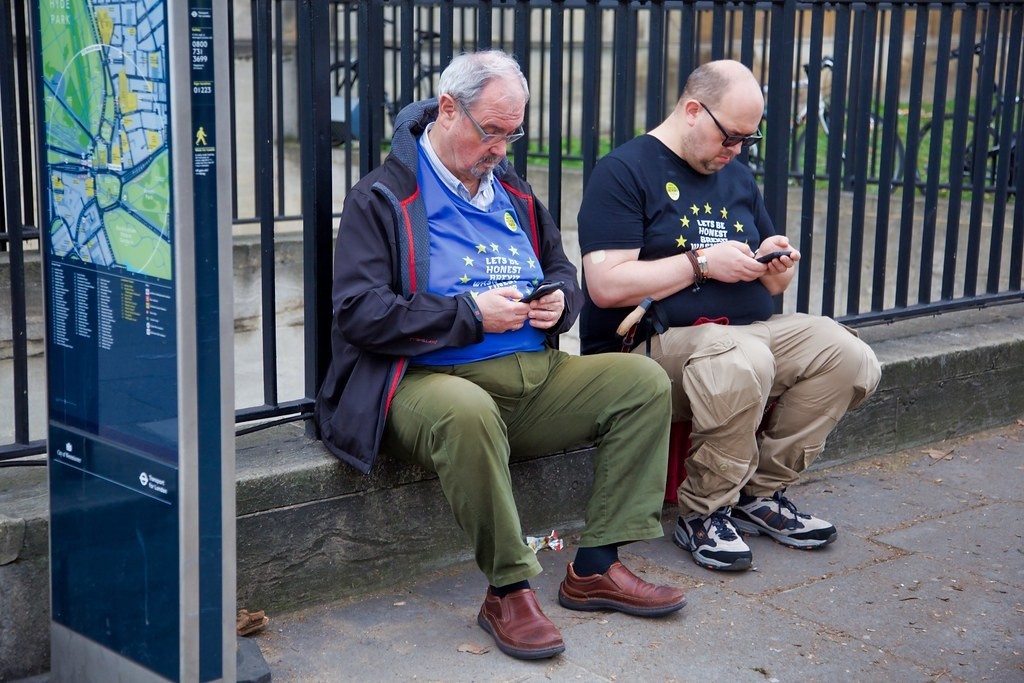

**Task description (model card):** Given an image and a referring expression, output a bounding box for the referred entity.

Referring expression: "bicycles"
[915,39,1024,198]
[745,56,904,194]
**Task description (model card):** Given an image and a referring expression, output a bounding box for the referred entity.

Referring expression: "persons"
[316,51,688,661]
[577,60,882,572]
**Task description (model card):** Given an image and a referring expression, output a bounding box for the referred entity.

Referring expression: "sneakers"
[672,505,753,571]
[731,490,837,550]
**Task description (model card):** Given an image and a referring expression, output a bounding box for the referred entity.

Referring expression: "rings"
[545,311,555,321]
[513,322,518,332]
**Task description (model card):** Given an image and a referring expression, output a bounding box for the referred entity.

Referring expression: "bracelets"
[684,248,709,290]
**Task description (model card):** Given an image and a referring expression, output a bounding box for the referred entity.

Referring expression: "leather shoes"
[477,588,565,660]
[559,559,688,617]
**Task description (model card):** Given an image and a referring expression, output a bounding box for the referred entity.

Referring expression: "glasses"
[457,100,524,144]
[700,102,763,147]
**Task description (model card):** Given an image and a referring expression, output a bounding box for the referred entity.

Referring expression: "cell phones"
[518,279,565,303]
[757,251,792,263]
